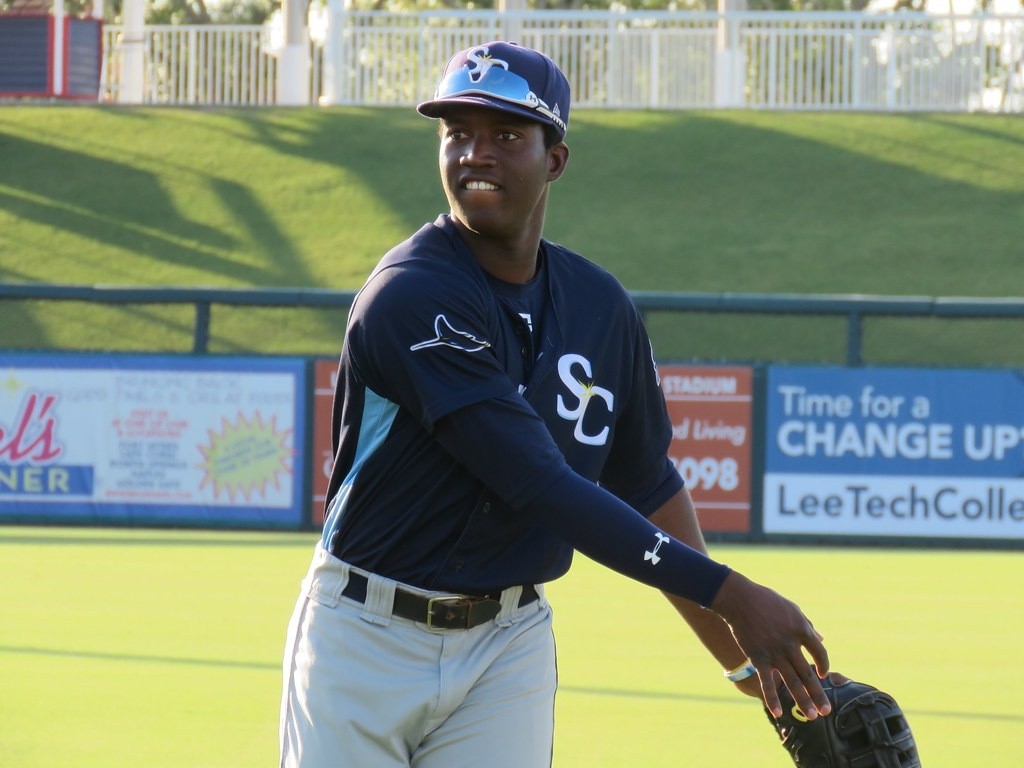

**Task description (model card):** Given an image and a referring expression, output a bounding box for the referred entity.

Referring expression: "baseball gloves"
[763,663,924,768]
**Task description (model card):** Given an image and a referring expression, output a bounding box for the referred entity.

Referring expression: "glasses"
[431,66,569,134]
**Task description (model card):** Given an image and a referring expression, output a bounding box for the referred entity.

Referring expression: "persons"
[280,42,830,768]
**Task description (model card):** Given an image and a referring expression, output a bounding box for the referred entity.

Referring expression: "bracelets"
[723,660,756,682]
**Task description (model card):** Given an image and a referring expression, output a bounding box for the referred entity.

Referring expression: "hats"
[416,41,570,149]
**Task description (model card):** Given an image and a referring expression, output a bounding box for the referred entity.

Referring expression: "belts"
[341,570,541,631]
